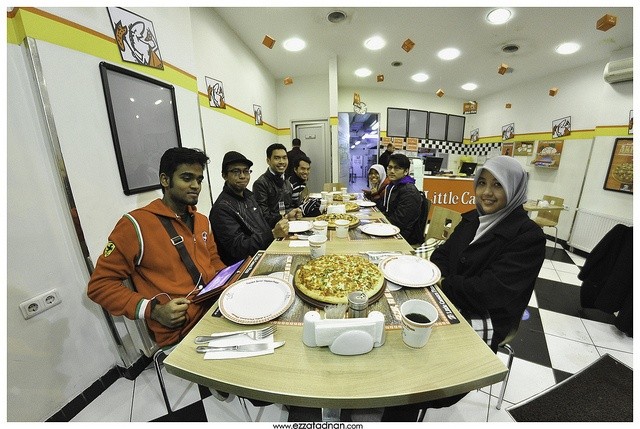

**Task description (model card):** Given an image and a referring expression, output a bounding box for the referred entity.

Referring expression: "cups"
[335,219,350,238]
[313,221,328,236]
[321,192,328,199]
[308,234,327,261]
[341,188,347,193]
[400,299,438,350]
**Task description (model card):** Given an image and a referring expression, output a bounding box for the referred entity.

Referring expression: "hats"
[222,151,253,167]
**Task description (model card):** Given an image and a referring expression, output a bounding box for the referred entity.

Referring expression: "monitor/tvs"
[459,162,477,177]
[424,157,444,176]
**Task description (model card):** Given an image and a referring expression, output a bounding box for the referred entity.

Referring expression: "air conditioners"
[603,57,633,84]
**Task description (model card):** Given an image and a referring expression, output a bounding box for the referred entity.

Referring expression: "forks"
[278,201,285,219]
[194,323,277,344]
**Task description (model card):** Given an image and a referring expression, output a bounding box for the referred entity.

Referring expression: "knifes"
[197,341,286,353]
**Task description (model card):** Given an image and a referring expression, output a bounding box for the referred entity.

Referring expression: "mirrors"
[338,112,382,192]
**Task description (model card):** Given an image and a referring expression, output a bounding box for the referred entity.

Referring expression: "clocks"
[353,102,367,115]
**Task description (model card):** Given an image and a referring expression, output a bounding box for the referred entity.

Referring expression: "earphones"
[198,285,204,290]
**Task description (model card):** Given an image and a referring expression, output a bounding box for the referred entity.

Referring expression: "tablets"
[193,255,252,305]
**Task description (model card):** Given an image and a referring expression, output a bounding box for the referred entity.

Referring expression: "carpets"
[503,352,633,421]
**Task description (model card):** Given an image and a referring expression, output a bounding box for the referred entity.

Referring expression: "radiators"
[565,207,632,253]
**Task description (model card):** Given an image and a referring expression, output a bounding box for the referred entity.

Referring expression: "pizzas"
[328,201,359,211]
[315,213,359,227]
[333,193,355,200]
[294,255,384,304]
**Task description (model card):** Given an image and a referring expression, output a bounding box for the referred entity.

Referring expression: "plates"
[288,221,313,233]
[361,223,400,236]
[357,202,376,206]
[309,196,321,199]
[313,220,361,230]
[378,254,442,288]
[324,208,360,212]
[293,278,387,311]
[219,275,295,325]
[333,197,357,201]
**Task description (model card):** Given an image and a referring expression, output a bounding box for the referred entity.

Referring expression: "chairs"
[409,204,462,260]
[108,269,258,421]
[404,197,432,249]
[551,116,572,138]
[470,129,479,142]
[527,194,564,249]
[418,249,546,421]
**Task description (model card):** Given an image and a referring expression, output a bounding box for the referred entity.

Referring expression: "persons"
[286,155,311,207]
[377,154,423,242]
[87,147,229,401]
[380,155,546,422]
[209,151,302,267]
[285,138,307,180]
[378,142,396,172]
[361,164,392,204]
[252,143,294,229]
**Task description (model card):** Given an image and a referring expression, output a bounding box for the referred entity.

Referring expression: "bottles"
[348,291,368,318]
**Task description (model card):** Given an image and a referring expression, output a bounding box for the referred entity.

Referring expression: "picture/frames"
[501,123,514,140]
[252,104,263,126]
[628,110,633,134]
[204,76,226,109]
[603,136,633,194]
[107,7,165,70]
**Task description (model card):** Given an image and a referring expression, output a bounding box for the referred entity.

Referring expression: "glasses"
[386,165,403,170]
[227,168,252,175]
